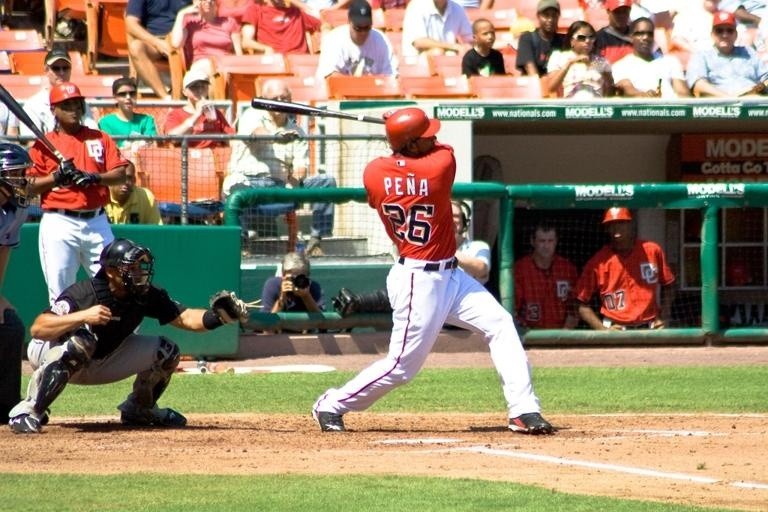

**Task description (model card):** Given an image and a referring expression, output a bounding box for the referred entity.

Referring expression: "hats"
[182,70,212,90]
[725,258,753,287]
[42,47,73,66]
[605,0,633,11]
[348,0,375,25]
[536,1,562,14]
[601,206,634,226]
[712,11,737,29]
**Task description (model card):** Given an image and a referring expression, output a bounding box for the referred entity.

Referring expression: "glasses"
[48,65,71,72]
[115,91,137,97]
[572,33,595,42]
[632,31,654,39]
[712,28,737,34]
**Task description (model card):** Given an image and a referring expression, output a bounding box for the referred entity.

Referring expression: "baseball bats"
[251,98,387,126]
[1,86,66,164]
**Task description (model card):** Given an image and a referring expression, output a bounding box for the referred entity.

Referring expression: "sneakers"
[508,412,553,435]
[310,407,346,434]
[119,407,188,428]
[306,246,326,257]
[8,414,41,436]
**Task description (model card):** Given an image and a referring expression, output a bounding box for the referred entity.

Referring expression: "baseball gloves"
[203,290,252,331]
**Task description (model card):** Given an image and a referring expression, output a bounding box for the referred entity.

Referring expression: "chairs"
[315,1,758,102]
[2,1,320,254]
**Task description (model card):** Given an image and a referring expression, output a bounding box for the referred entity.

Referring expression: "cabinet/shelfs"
[662,201,767,292]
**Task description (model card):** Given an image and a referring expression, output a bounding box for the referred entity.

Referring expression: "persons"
[9,239,247,435]
[311,107,555,434]
[0,143,39,424]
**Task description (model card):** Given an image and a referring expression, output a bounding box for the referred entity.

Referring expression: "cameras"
[291,271,311,290]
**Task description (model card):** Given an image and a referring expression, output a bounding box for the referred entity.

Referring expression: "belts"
[50,206,105,219]
[612,321,650,330]
[398,257,458,271]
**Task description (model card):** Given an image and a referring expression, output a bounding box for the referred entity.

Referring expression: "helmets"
[0,143,35,209]
[385,108,441,151]
[49,81,87,105]
[99,236,157,297]
